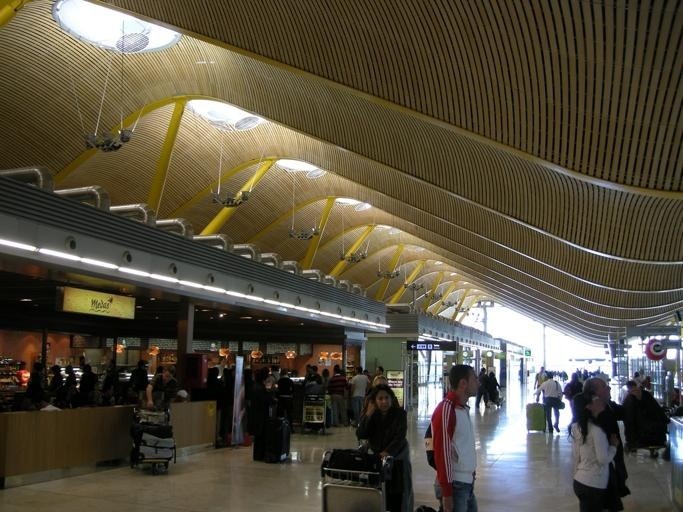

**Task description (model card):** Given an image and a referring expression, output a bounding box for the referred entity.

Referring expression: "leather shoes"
[549,426,560,432]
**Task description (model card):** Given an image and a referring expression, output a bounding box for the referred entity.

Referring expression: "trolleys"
[131,407,173,470]
[301,396,327,435]
[319,448,394,512]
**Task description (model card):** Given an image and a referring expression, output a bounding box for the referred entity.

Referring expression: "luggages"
[326,405,331,428]
[268,407,291,463]
[527,403,546,432]
[485,380,492,388]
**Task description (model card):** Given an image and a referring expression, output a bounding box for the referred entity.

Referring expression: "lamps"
[334,197,376,264]
[377,225,476,313]
[189,99,273,210]
[54,1,179,154]
[276,158,324,241]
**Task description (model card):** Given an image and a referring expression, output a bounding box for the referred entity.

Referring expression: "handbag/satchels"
[557,398,565,409]
[564,383,573,400]
[130,398,175,459]
[328,449,393,484]
[609,461,631,498]
[306,395,324,405]
[424,422,437,471]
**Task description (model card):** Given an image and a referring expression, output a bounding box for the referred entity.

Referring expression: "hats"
[178,390,187,398]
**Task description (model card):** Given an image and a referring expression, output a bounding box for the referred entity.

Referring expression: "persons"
[487,371,499,404]
[475,367,490,408]
[429,364,481,511]
[23,360,236,423]
[355,384,414,512]
[244,363,387,435]
[580,378,643,512]
[567,391,620,512]
[532,365,682,460]
